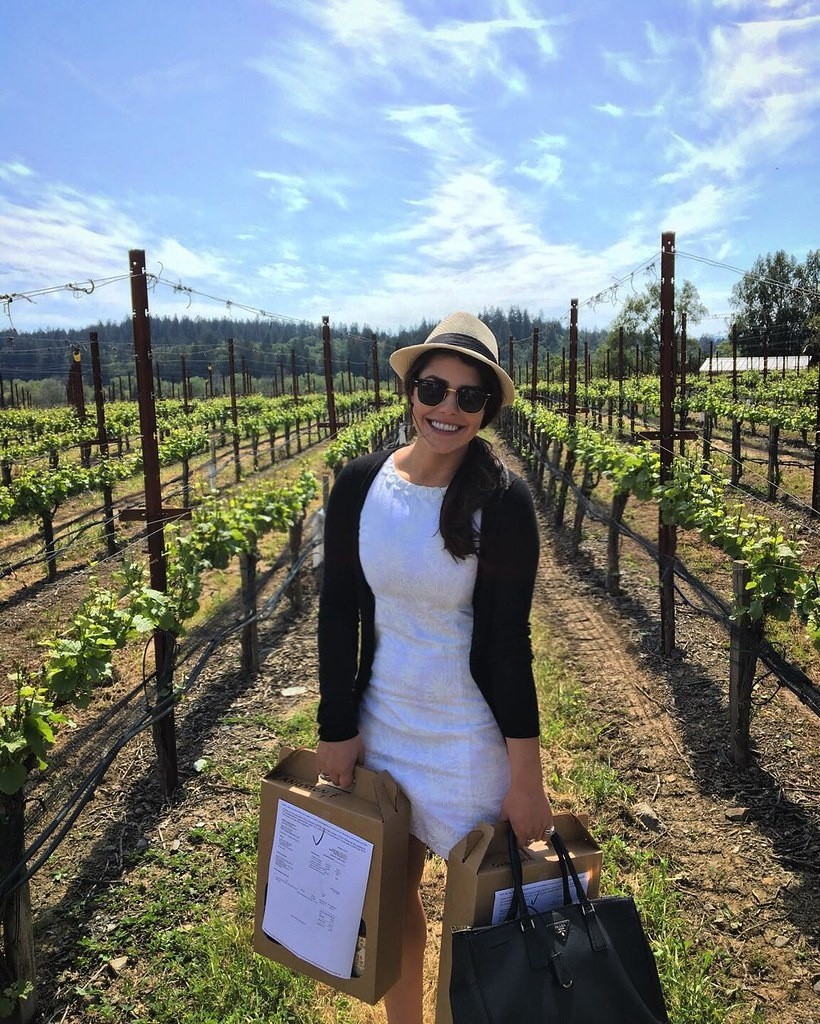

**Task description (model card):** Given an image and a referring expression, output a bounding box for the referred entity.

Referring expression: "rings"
[544,826,555,835]
[320,771,326,779]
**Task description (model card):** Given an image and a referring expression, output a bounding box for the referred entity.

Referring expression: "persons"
[316,313,555,1024]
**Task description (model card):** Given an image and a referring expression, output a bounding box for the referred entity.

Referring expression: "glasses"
[411,377,492,415]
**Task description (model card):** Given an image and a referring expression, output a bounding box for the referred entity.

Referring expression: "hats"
[387,312,516,409]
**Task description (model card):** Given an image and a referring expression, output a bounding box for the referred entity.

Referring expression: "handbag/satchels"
[450,822,669,1024]
